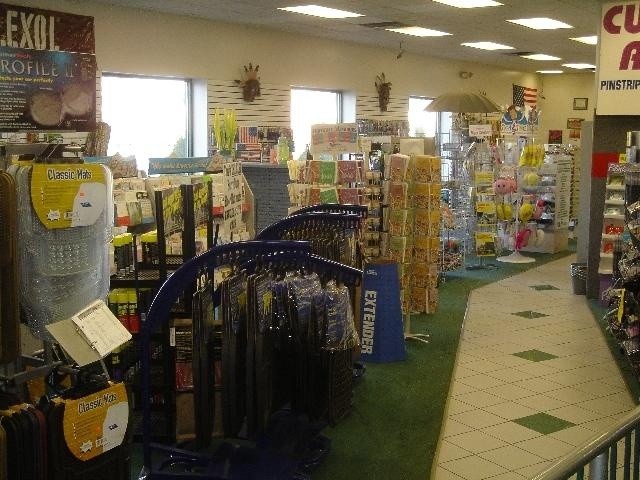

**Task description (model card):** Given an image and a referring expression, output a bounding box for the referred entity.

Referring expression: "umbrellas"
[424,88,501,113]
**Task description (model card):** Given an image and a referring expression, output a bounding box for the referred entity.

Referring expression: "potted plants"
[214,106,237,157]
[238,62,261,101]
[376,71,393,112]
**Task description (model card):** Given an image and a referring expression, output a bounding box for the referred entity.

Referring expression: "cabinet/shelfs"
[108,180,189,341]
[380,153,439,342]
[445,143,514,259]
[599,130,640,308]
[298,123,380,261]
[148,157,296,236]
[518,142,579,255]
[136,179,214,276]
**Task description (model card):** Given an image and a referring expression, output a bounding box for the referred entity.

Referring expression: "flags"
[512,84,538,107]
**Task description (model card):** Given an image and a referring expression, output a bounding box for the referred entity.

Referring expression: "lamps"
[561,63,596,70]
[385,26,455,38]
[504,17,576,31]
[460,41,517,51]
[535,69,564,74]
[431,0,505,9]
[276,5,367,20]
[568,35,597,46]
[519,53,563,61]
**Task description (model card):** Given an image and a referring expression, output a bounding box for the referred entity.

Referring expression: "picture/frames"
[567,118,586,129]
[548,129,562,144]
[569,129,580,140]
[573,97,588,110]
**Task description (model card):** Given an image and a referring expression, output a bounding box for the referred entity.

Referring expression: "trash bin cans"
[571,263,588,295]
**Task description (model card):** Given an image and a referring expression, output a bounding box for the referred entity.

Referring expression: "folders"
[45,297,133,368]
